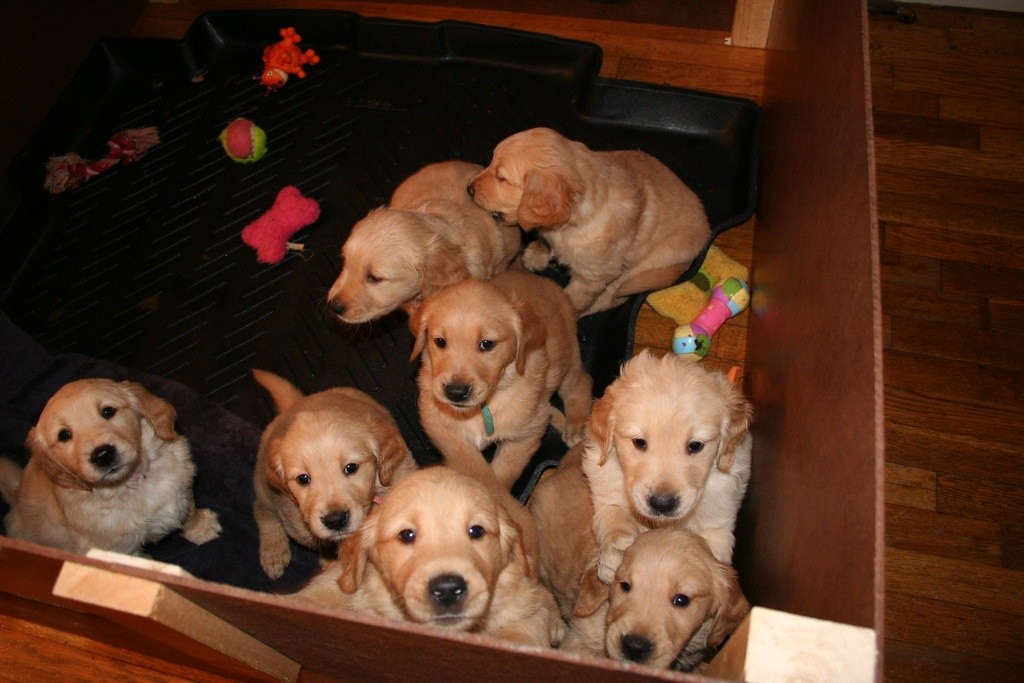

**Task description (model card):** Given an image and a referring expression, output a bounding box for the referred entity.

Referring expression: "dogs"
[279,465,567,647]
[0,377,224,558]
[409,270,592,479]
[253,369,421,579]
[325,160,522,325]
[529,349,754,670]
[467,126,710,321]
